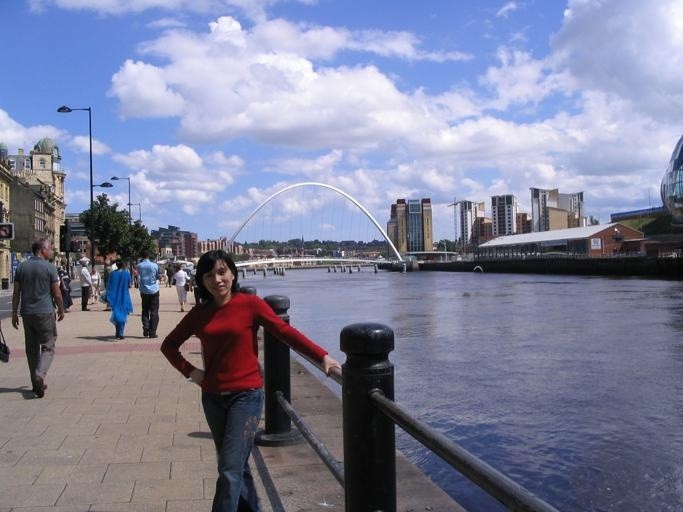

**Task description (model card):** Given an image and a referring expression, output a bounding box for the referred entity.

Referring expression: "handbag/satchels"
[185,284,189,291]
[0,342,8,363]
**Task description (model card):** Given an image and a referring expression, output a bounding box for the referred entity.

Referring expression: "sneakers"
[143,328,158,338]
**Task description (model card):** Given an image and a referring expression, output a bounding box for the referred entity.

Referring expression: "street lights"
[58,106,113,274]
[110,177,132,285]
[127,203,142,227]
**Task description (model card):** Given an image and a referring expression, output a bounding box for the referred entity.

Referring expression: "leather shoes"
[32,376,47,398]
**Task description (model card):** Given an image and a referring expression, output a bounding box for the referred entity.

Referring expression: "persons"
[136,248,161,338]
[105,260,134,340]
[49,256,200,313]
[160,250,341,512]
[12,238,64,398]
